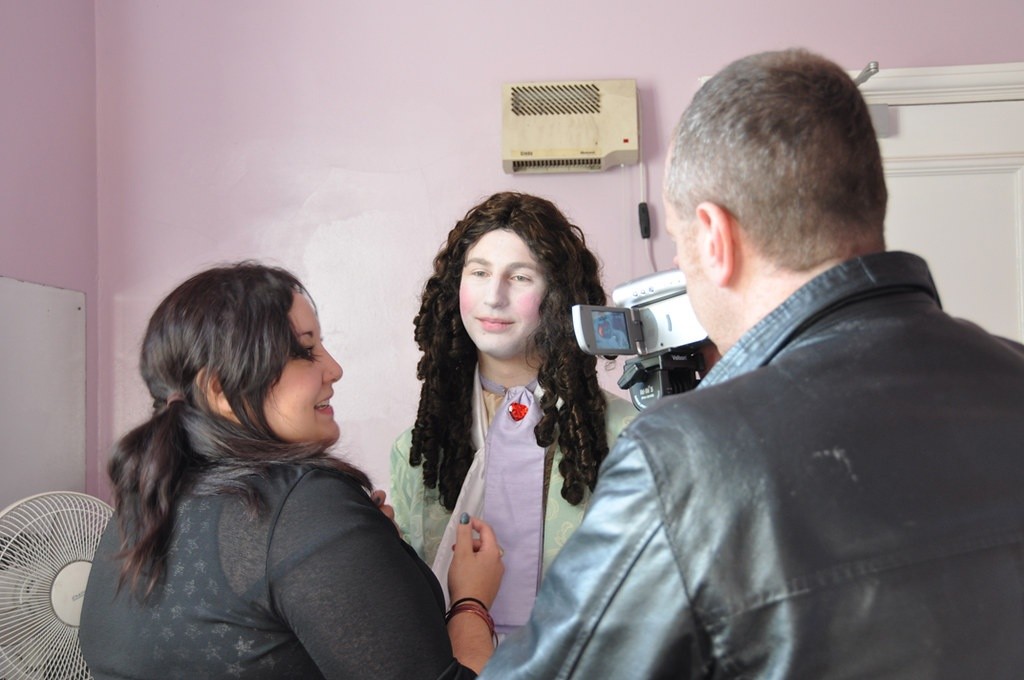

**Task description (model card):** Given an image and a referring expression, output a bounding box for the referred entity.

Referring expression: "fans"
[1,492,115,680]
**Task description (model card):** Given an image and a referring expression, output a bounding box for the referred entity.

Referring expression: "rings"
[498,546,504,557]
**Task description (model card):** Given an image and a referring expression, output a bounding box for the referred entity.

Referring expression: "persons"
[78,257,505,679]
[386,190,641,645]
[472,44,1024,680]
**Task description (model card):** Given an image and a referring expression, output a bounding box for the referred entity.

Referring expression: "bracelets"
[443,597,499,648]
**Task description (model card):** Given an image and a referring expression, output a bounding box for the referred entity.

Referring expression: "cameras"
[572,270,712,415]
